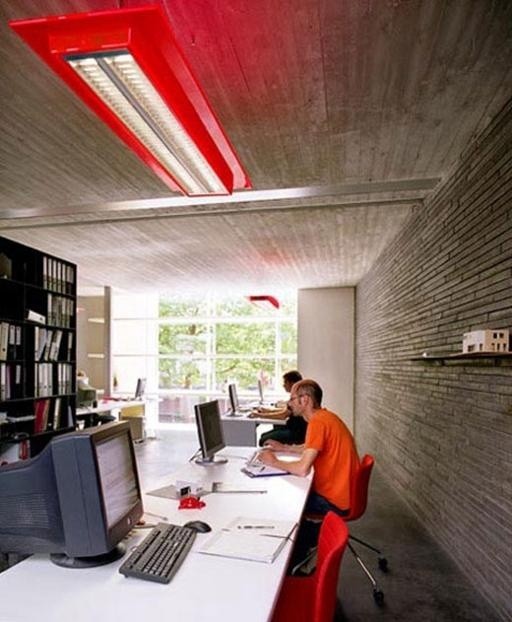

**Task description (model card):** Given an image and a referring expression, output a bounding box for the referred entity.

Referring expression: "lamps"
[248,296,282,314]
[7,3,252,203]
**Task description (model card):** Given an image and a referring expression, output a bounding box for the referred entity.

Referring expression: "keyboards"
[119,521,198,584]
[249,451,267,467]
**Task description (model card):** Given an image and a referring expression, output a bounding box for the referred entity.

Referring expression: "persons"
[256,377,362,576]
[247,370,308,447]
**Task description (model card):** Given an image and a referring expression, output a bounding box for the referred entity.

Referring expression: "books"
[247,450,303,475]
[0,251,80,468]
[193,510,298,566]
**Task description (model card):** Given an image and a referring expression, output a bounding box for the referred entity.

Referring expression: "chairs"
[266,512,349,622]
[290,452,392,604]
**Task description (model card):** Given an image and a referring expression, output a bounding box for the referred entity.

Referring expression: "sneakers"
[300,546,318,574]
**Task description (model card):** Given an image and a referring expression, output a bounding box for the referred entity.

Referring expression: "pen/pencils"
[259,466,266,473]
[238,525,274,529]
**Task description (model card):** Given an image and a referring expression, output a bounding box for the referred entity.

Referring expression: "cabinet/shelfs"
[1,235,77,468]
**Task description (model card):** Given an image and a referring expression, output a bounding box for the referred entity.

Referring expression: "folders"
[0,322,22,361]
[1,363,27,400]
[23,257,74,297]
[25,293,75,328]
[34,326,73,362]
[35,363,73,398]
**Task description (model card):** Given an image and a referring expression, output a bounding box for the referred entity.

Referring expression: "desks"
[75,391,158,440]
[1,442,314,622]
[219,400,292,448]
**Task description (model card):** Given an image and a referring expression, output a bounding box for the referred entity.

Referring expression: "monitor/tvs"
[256,379,267,405]
[78,376,97,408]
[227,383,243,416]
[0,420,144,568]
[194,400,228,464]
[135,377,148,401]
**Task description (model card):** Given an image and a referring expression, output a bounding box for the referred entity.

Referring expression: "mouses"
[263,444,274,450]
[184,521,212,532]
